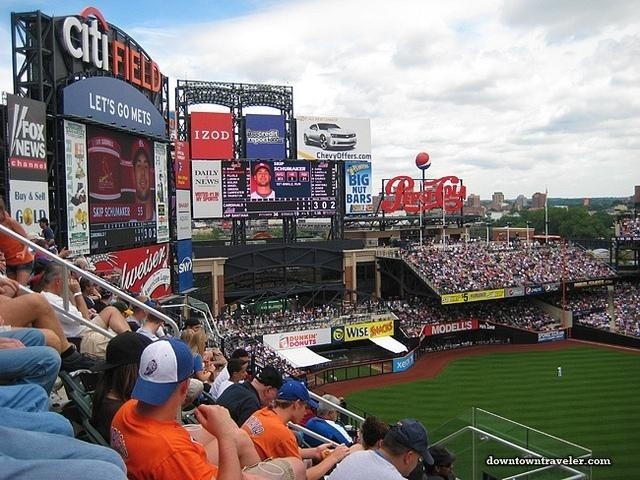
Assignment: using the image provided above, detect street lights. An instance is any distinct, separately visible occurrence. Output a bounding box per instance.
[486,222,493,247]
[526,221,532,248]
[465,222,471,246]
[419,224,426,248]
[506,222,513,248]
[544,219,552,244]
[442,225,448,251]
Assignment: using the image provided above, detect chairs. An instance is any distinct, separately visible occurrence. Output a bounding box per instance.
[53,364,201,451]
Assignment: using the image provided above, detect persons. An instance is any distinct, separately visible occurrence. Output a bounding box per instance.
[0,196,640,480]
[131,138,156,220]
[252,163,276,201]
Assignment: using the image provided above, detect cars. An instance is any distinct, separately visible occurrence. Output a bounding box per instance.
[303,122,357,151]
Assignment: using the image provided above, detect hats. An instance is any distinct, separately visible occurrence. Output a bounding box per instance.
[255,366,319,409]
[390,416,434,466]
[132,297,156,311]
[253,163,271,174]
[427,445,456,464]
[38,217,48,223]
[88,330,154,370]
[131,337,204,407]
[132,138,152,166]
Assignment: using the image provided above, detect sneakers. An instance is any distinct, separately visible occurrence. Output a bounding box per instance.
[63,352,108,372]
[49,376,73,411]
